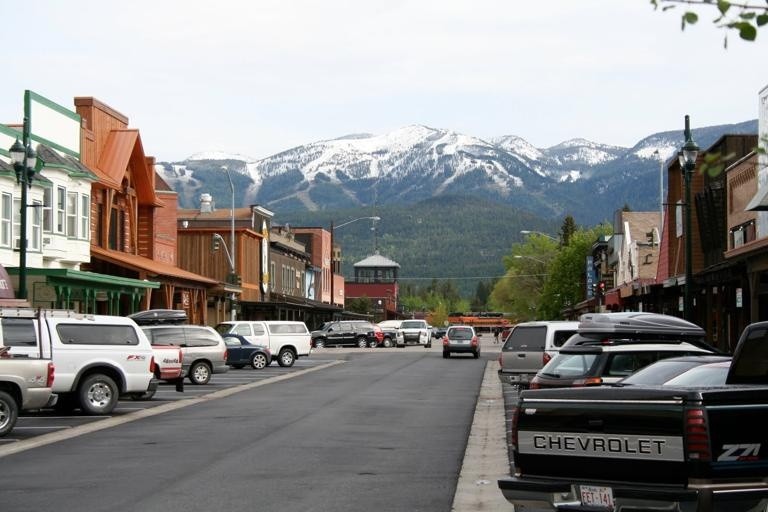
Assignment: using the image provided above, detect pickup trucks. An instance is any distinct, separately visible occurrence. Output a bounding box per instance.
[131,344,186,403]
[497,318,767,512]
[0,357,58,438]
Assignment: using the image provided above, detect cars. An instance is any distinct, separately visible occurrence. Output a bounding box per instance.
[441,325,484,359]
[556,334,632,379]
[661,361,730,389]
[584,356,732,390]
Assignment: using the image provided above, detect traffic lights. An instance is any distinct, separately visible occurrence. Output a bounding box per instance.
[592,283,598,296]
[599,281,607,297]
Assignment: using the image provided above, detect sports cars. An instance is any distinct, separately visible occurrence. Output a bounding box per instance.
[220,333,271,370]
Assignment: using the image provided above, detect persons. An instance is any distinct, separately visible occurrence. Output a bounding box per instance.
[492,328,500,344]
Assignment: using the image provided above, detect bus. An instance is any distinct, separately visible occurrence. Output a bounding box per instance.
[446,312,519,333]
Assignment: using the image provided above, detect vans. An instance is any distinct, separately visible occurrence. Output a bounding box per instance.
[497,320,580,387]
[501,325,513,342]
[126,309,232,386]
[213,321,312,368]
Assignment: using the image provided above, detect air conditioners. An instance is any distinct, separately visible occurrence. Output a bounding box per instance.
[12,237,29,251]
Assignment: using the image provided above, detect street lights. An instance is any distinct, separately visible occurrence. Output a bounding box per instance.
[511,251,546,267]
[518,228,562,247]
[329,215,382,306]
[8,116,47,298]
[211,164,240,321]
[673,111,699,315]
[652,147,666,243]
[373,299,381,322]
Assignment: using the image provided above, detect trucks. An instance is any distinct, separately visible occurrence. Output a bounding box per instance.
[0,297,158,416]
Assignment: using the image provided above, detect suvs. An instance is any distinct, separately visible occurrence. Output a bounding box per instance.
[310,318,447,351]
[524,311,724,393]
